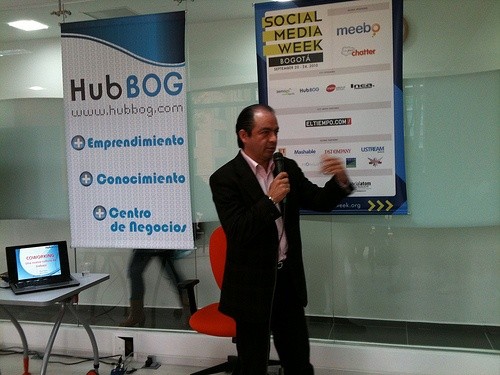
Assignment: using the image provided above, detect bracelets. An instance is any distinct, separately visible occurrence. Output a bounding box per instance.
[269,196,276,206]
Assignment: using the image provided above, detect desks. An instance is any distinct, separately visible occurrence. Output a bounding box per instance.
[0,273,110,375]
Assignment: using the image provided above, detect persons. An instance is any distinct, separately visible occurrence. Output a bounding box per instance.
[118,249,200,327]
[208,102,356,375]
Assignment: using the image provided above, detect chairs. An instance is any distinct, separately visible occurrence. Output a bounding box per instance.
[176,226,284,375]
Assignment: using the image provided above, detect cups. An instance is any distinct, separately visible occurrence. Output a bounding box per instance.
[79,263,90,277]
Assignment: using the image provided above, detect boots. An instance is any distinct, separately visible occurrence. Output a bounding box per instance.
[120,300,146,326]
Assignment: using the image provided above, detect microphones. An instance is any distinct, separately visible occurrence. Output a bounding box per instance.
[273,152,286,203]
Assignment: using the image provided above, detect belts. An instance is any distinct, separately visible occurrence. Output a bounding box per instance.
[278,259,288,269]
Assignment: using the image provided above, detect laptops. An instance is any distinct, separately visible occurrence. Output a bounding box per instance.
[6,241,80,295]
